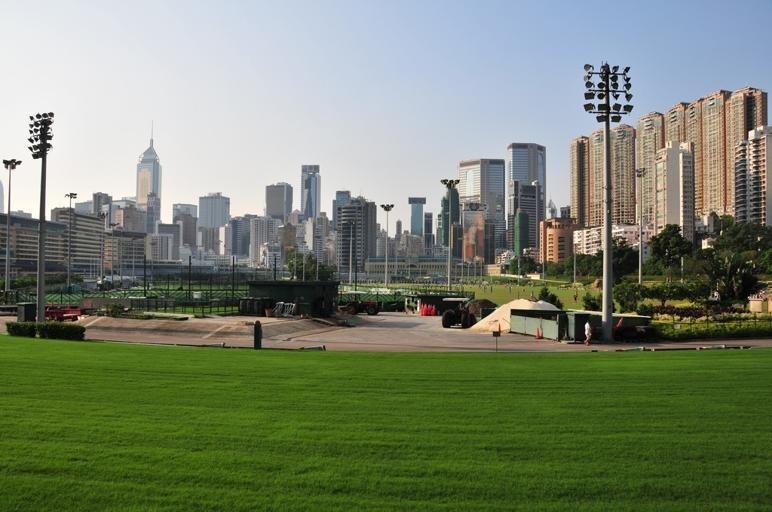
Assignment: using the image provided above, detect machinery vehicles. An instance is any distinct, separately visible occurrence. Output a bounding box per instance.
[336,293,383,315]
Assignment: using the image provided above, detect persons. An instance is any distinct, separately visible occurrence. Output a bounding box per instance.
[584,320,593,346]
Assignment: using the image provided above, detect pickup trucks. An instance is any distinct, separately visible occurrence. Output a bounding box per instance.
[45,304,80,321]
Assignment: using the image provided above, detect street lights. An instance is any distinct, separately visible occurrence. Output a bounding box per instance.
[346,218,356,283]
[440,178,460,290]
[583,61,633,342]
[379,203,394,289]
[64,193,76,286]
[97,211,109,280]
[3,158,22,293]
[27,112,54,322]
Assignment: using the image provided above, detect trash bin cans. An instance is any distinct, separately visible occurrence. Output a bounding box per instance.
[17,301,37,322]
